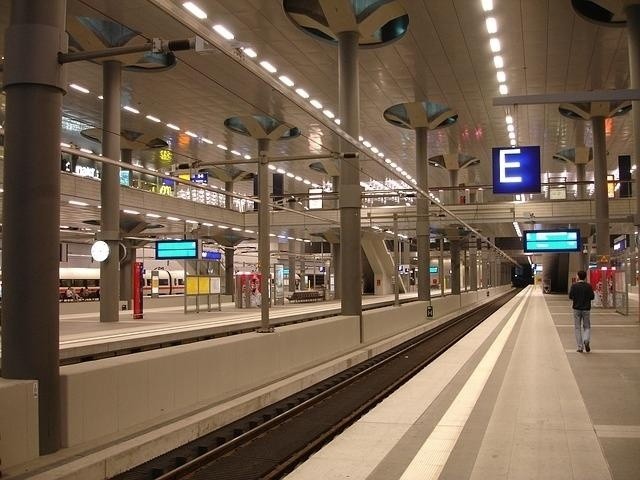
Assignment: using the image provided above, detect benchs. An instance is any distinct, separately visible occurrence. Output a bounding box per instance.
[286,292,318,303]
[61,291,100,303]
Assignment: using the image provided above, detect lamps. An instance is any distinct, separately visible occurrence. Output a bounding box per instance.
[120,243,128,263]
[90,241,111,262]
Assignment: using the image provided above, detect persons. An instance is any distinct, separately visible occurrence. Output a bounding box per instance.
[66,286,100,302]
[68,142,80,172]
[569,271,594,353]
[61,155,67,172]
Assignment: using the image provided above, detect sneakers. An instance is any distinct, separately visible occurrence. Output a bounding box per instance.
[585,340,590,352]
[577,350,583,352]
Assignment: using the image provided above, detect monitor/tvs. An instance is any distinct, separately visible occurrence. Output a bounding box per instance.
[155,239,198,260]
[429,267,438,273]
[120,170,129,186]
[523,229,580,255]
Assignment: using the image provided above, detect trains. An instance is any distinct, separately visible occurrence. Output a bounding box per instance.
[59,267,184,297]
